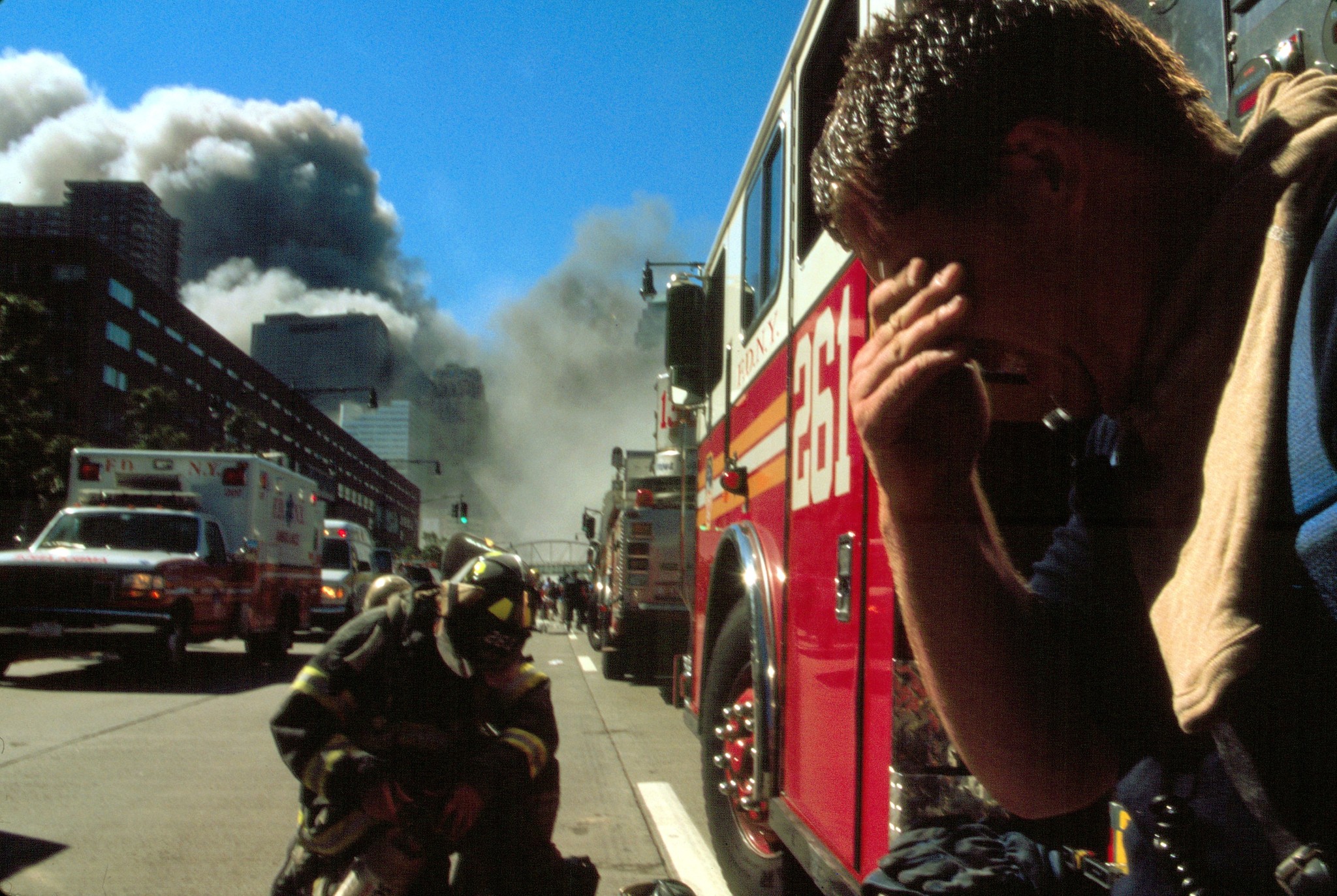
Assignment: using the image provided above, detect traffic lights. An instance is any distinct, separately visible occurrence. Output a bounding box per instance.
[452,505,459,518]
[460,502,468,523]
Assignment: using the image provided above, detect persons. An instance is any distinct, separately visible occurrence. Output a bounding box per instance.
[524,569,590,632]
[809,0,1337,896]
[269,550,560,896]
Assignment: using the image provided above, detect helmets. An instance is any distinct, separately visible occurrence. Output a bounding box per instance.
[437,549,540,678]
[363,574,411,609]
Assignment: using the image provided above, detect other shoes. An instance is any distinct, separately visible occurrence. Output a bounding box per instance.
[455,856,599,895]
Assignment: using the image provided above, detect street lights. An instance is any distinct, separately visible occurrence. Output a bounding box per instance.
[288,387,378,473]
[379,457,442,547]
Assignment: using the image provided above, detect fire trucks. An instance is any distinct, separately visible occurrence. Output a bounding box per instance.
[0,446,324,687]
[660,1,1337,896]
[587,446,697,681]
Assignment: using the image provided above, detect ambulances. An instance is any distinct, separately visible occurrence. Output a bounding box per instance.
[311,518,378,629]
